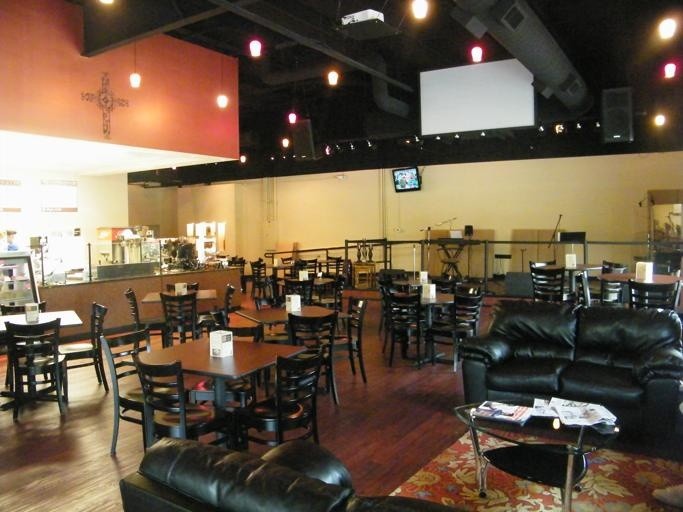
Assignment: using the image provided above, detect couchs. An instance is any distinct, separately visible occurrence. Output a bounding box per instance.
[112,437,468,511]
[456,296,683,457]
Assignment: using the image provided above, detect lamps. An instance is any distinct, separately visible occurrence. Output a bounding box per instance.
[129,39,143,90]
[216,4,228,109]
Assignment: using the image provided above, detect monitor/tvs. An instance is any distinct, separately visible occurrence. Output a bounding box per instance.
[392,166,421,192]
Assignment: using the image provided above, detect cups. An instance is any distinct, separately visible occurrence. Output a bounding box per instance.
[273,258,282,267]
[142,225,149,237]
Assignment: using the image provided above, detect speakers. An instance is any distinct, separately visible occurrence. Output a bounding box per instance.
[290,118,328,161]
[602,86,634,144]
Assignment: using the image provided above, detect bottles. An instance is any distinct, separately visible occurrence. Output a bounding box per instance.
[182,260,190,270]
[205,226,211,237]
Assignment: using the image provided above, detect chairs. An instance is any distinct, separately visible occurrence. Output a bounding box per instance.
[96,321,185,457]
[59,301,112,392]
[286,311,342,405]
[124,225,683,371]
[3,301,49,388]
[322,295,370,381]
[129,360,213,455]
[224,344,326,451]
[3,319,69,422]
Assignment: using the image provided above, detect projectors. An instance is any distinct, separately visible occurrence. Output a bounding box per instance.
[341,8,384,25]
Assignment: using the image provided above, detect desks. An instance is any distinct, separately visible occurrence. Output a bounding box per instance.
[3,310,85,410]
[137,338,307,416]
[451,396,619,511]
